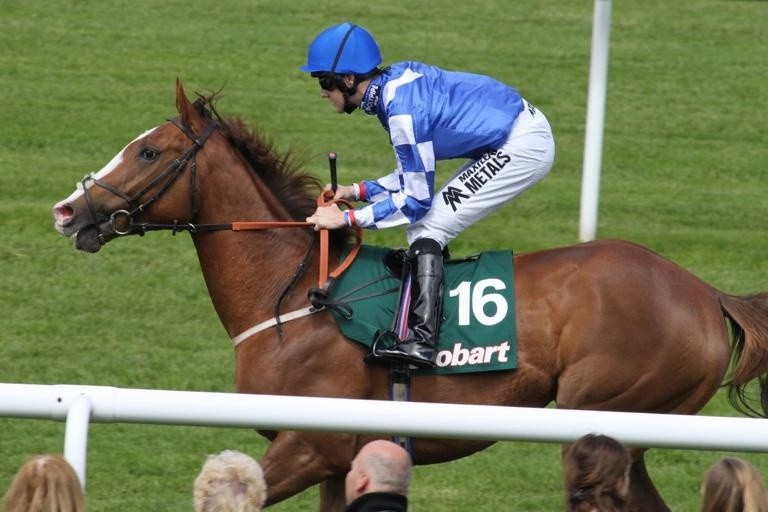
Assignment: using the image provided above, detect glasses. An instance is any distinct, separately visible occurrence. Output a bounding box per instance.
[320,25,352,90]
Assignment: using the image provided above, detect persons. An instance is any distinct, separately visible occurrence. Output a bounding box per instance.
[341,438,412,512]
[2,457,86,512]
[563,433,634,512]
[192,449,268,512]
[298,22,555,371]
[700,457,768,512]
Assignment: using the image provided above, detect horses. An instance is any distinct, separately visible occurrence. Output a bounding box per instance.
[49,74,768,512]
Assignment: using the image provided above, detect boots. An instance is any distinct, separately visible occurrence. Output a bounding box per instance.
[364,238,443,365]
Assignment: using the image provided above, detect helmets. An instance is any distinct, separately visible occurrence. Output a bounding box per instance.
[300,25,381,73]
